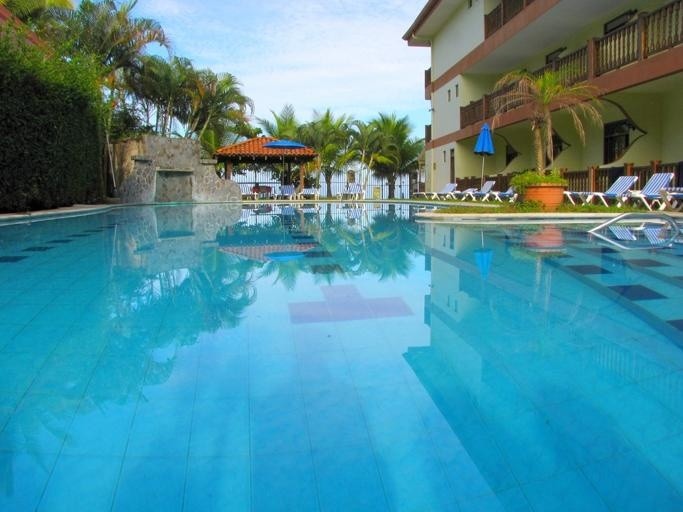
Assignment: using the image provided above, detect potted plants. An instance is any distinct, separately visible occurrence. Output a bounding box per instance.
[509,168,569,212]
[506,223,566,259]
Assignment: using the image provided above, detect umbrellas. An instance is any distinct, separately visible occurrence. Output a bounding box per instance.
[262,205,308,265]
[262,139,304,201]
[472,223,494,286]
[472,123,496,188]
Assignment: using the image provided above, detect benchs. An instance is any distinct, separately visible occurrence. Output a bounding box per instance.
[242,193,258,200]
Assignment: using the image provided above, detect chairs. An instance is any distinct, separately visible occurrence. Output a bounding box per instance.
[607,224,683,247]
[412,180,518,203]
[279,182,363,200]
[279,204,364,219]
[563,172,683,212]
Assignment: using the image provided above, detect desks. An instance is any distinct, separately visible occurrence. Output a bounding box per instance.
[253,204,273,214]
[251,186,271,199]
